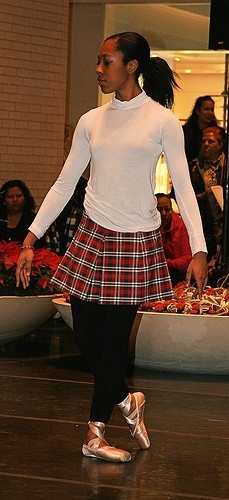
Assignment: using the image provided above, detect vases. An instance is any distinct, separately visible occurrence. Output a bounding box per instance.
[0,293,61,345]
[52,297,229,376]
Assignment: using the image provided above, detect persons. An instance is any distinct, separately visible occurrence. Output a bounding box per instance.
[14,31,207,463]
[0,179,47,287]
[179,94,225,173]
[152,192,193,288]
[185,122,229,276]
[43,176,86,259]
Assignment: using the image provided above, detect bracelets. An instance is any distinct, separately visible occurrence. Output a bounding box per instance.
[20,246,34,250]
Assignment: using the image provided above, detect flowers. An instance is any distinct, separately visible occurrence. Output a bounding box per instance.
[63,282,229,316]
[0,239,63,296]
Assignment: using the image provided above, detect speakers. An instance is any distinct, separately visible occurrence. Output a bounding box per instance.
[208,0,229,51]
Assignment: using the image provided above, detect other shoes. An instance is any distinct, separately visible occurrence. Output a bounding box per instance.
[82,421,132,461]
[118,391,150,449]
